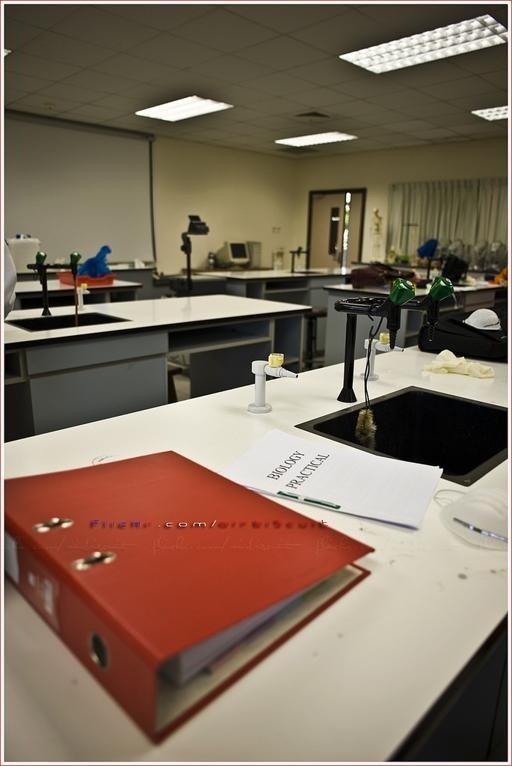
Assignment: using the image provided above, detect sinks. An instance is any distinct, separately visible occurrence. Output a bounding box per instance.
[3,310,135,332]
[294,384,508,488]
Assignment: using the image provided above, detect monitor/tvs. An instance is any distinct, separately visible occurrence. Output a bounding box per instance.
[215,241,250,270]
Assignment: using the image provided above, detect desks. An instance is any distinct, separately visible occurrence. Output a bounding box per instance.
[321,280,505,369]
[14,278,143,308]
[2,294,312,444]
[4,351,510,766]
[192,266,354,348]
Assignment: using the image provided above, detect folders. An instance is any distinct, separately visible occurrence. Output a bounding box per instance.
[4,451,375,749]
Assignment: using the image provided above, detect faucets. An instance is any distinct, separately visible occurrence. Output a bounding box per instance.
[330,273,457,403]
[24,249,87,316]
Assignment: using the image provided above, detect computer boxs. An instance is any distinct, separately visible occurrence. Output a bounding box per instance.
[247,240,262,269]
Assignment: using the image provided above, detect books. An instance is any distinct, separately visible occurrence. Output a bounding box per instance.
[229,427,444,529]
